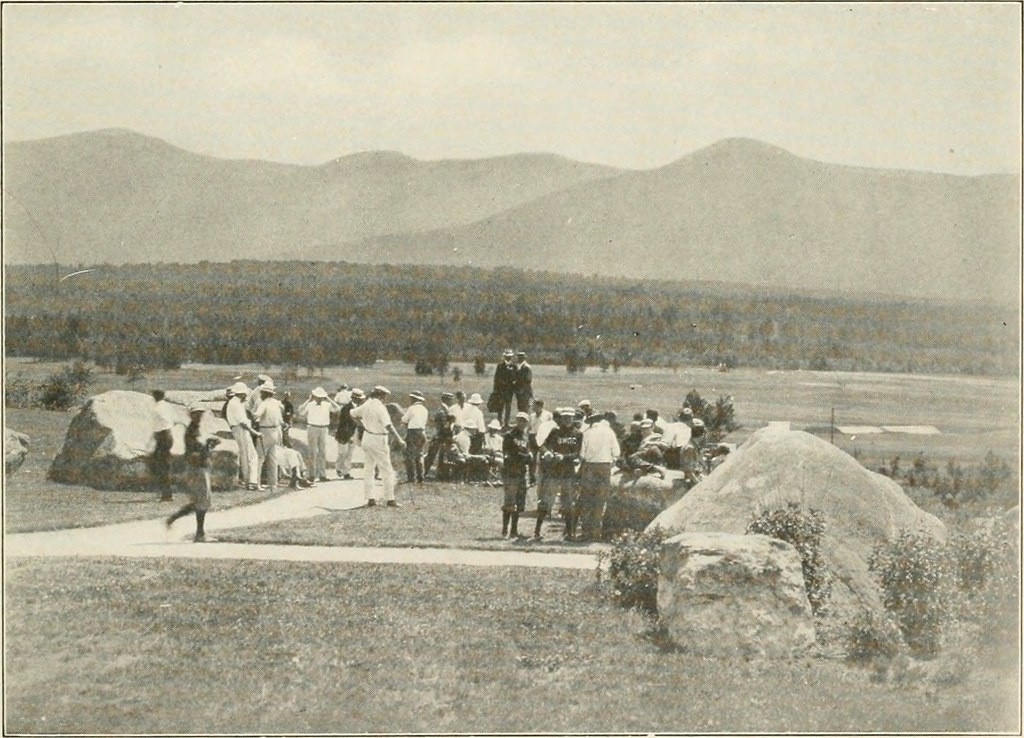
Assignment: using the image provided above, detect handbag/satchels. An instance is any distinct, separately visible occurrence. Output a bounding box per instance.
[540,455,562,478]
[487,391,504,412]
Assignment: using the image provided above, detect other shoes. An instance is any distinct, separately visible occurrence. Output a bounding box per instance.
[369,499,376,505]
[388,501,402,507]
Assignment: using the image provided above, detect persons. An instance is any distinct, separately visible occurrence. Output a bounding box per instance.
[221,371,733,541]
[146,388,176,503]
[493,348,520,427]
[166,401,222,544]
[513,351,533,415]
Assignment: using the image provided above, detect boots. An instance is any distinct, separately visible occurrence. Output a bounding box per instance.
[502,510,510,536]
[194,511,217,542]
[510,511,519,536]
[534,511,548,540]
[166,504,194,529]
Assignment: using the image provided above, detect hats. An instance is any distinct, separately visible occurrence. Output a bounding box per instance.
[488,419,502,430]
[578,400,590,406]
[464,418,476,429]
[560,407,575,416]
[516,412,529,421]
[234,383,247,393]
[584,408,604,424]
[312,387,327,397]
[503,351,514,356]
[468,394,483,404]
[350,389,366,398]
[260,385,276,395]
[640,419,653,427]
[409,390,425,400]
[516,352,527,358]
[188,402,205,412]
[373,386,391,394]
[442,393,453,399]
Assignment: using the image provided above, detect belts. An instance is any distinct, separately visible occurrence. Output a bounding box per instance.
[259,426,276,428]
[311,424,328,428]
[365,429,388,435]
